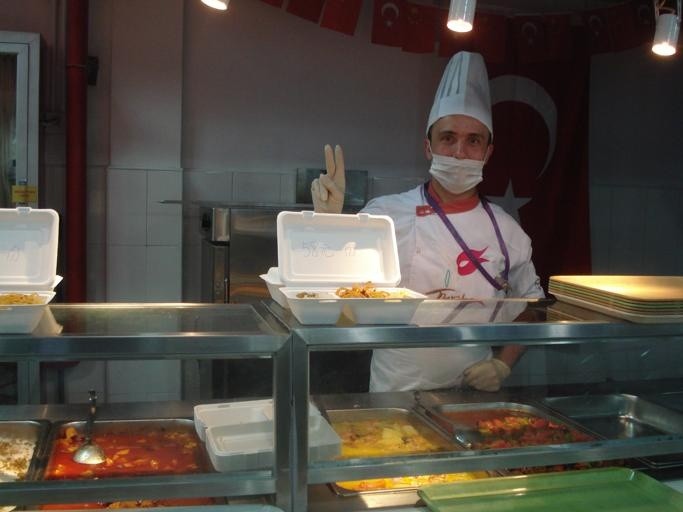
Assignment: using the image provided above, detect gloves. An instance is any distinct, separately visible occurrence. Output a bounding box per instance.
[463,357,512,394]
[311,143,346,214]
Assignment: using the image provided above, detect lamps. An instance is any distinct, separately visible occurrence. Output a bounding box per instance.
[650,0,681,62]
[445,1,476,34]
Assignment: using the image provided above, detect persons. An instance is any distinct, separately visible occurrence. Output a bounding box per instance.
[311,115,546,392]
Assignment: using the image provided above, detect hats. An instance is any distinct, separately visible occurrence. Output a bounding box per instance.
[424,50,494,145]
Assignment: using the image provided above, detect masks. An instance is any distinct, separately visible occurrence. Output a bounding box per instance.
[427,139,490,196]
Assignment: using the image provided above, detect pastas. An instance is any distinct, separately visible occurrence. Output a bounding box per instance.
[0,292,44,304]
[335,284,390,298]
